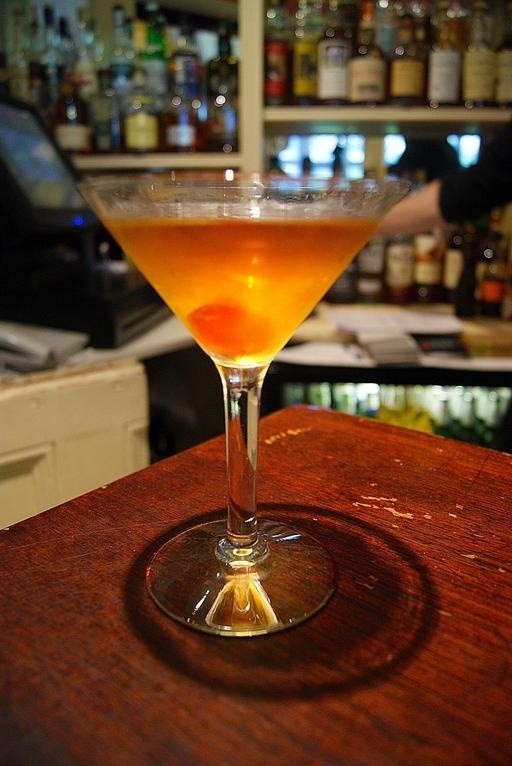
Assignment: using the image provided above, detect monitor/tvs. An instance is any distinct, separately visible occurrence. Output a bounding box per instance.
[0,91,102,242]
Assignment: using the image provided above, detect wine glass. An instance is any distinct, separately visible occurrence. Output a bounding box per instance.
[77,178,412,642]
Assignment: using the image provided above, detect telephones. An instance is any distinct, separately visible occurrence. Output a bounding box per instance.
[1,320,90,375]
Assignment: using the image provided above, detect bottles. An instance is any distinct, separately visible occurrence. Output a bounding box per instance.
[434,388,506,444]
[263,0,512,109]
[325,209,511,322]
[266,146,429,183]
[11,0,240,153]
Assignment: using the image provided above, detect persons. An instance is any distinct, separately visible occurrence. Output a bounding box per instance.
[375,117,509,240]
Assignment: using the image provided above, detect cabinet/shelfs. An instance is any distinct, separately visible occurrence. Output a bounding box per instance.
[1,0,512,392]
[1,345,148,527]
